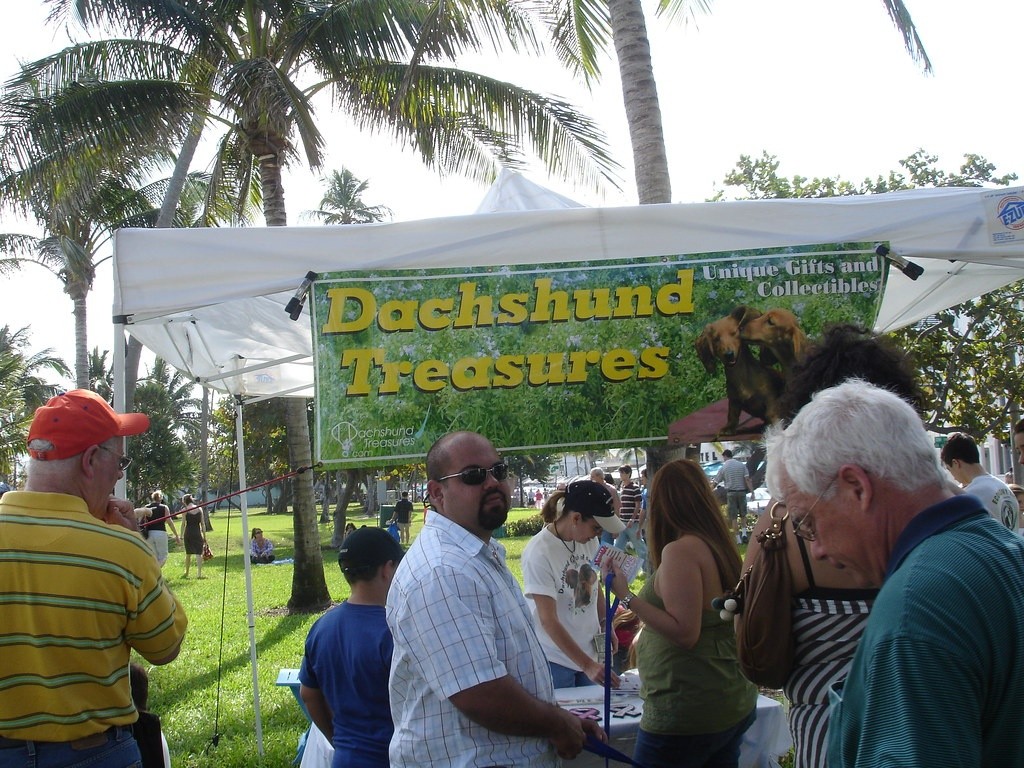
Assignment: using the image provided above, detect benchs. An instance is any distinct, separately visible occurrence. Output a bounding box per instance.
[276,669,313,762]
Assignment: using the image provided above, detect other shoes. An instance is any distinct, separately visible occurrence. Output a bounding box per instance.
[737,540,742,545]
[742,534,748,543]
[401,542,409,549]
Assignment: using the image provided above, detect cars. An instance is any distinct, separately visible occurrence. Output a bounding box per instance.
[512,452,771,518]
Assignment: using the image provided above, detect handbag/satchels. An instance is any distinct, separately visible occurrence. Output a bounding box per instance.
[736,501,793,689]
[139,504,148,539]
[203,542,213,560]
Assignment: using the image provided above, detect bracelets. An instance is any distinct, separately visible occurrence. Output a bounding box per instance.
[621,593,634,610]
[599,617,605,624]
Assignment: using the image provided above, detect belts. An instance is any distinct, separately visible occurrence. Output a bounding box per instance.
[728,490,746,492]
[0,725,128,749]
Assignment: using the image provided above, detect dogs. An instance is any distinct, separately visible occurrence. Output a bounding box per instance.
[693,304,818,436]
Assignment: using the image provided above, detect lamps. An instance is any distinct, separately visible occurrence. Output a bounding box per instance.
[875,243,924,281]
[285,270,317,321]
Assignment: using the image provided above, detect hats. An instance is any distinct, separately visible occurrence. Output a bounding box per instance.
[564,481,627,533]
[26,389,149,461]
[338,525,406,573]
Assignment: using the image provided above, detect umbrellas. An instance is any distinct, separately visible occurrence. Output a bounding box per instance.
[668,398,767,445]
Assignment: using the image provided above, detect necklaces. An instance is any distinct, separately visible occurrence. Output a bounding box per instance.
[555,523,575,558]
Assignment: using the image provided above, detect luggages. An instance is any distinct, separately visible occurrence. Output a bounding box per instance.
[386,519,400,544]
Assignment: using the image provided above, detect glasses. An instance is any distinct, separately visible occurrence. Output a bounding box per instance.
[423,464,509,502]
[81,444,133,470]
[255,531,263,534]
[793,468,869,542]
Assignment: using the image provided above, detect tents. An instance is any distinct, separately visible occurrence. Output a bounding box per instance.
[111,172,1024,750]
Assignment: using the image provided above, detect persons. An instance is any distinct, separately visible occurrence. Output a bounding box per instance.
[711,450,755,545]
[590,464,648,573]
[602,460,758,768]
[180,494,207,580]
[386,430,608,767]
[346,523,356,534]
[519,480,621,688]
[733,322,1024,768]
[130,664,172,768]
[528,488,549,509]
[134,491,180,568]
[298,525,406,768]
[686,448,699,461]
[0,388,189,768]
[391,492,413,544]
[251,528,275,563]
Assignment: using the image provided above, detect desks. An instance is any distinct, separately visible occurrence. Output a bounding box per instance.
[300,679,793,768]
